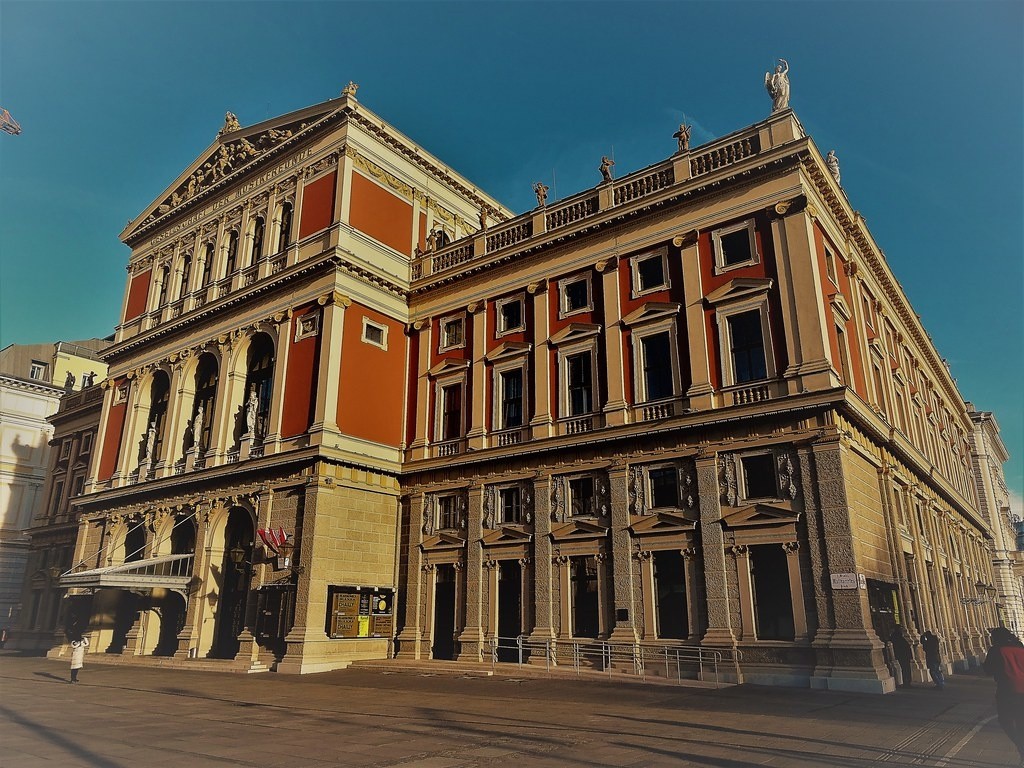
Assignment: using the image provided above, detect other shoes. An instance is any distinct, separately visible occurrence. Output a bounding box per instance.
[903,685,915,689]
[72,680,79,684]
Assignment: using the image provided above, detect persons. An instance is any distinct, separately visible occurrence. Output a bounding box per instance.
[890,624,912,688]
[828,149,840,187]
[477,207,490,229]
[0,629,8,649]
[673,125,690,150]
[246,392,259,433]
[772,58,790,108]
[920,631,944,688]
[87,371,96,385]
[983,628,1024,752]
[70,635,89,684]
[342,80,359,94]
[192,406,206,446]
[146,422,159,460]
[427,229,440,251]
[535,183,549,205]
[599,156,614,181]
